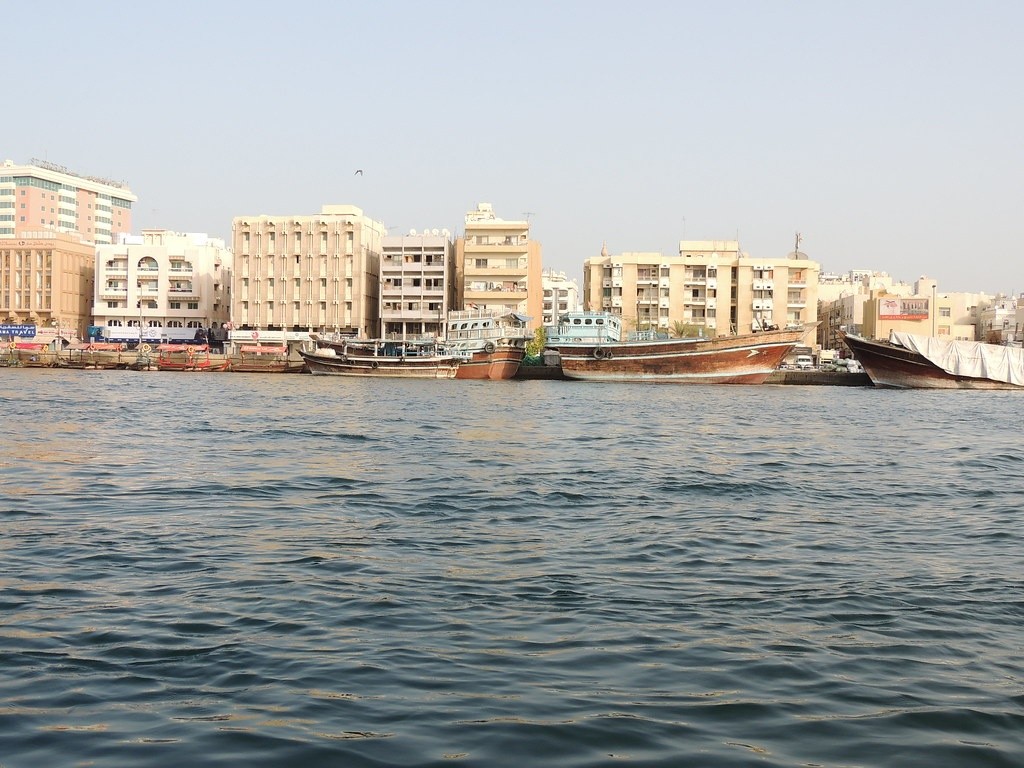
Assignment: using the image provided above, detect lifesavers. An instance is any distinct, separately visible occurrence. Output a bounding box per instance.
[341,354,348,362]
[606,352,613,358]
[350,360,356,365]
[592,347,606,360]
[9,342,16,349]
[90,343,95,349]
[188,346,194,353]
[400,357,405,363]
[43,344,49,351]
[143,344,152,352]
[484,341,496,354]
[371,361,379,369]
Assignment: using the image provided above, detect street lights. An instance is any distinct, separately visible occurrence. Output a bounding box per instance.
[932,283,937,336]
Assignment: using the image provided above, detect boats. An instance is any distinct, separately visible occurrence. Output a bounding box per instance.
[63,337,128,368]
[299,310,533,381]
[129,348,160,372]
[1,337,56,369]
[229,343,305,375]
[155,343,233,372]
[832,329,1024,391]
[540,311,824,386]
